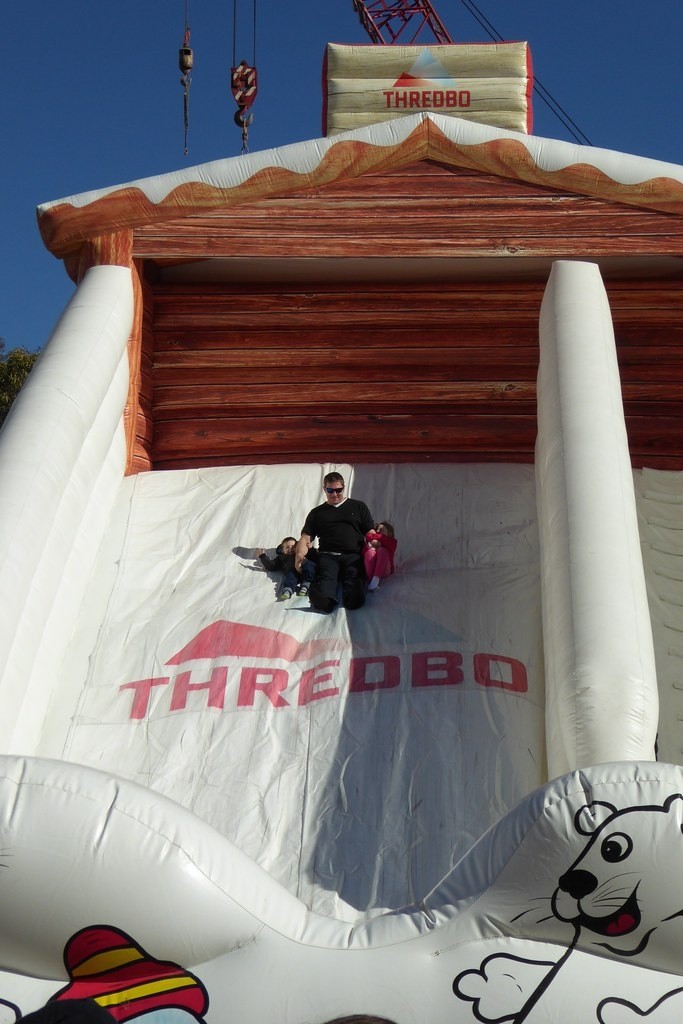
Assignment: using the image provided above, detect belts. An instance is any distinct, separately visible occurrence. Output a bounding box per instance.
[318,551,342,556]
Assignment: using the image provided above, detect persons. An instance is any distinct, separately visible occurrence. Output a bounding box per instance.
[362,521,397,593]
[294,472,379,613]
[255,537,319,600]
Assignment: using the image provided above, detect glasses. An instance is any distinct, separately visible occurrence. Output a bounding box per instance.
[326,487,343,493]
